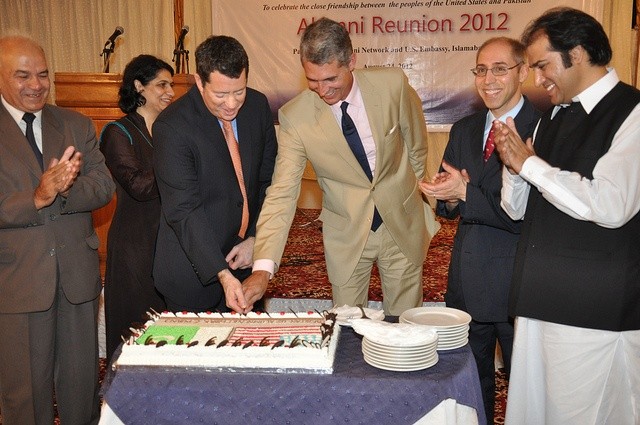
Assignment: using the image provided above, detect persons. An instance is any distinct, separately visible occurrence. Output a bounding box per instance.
[490,5,640,425]
[235,18,442,318]
[418,36,544,425]
[99,54,175,371]
[0,35,117,425]
[151,36,279,315]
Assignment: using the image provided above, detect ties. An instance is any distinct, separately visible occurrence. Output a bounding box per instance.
[483,121,496,162]
[340,102,383,232]
[221,121,249,238]
[23,113,43,171]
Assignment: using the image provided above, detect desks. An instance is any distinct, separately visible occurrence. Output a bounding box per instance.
[97,314,486,425]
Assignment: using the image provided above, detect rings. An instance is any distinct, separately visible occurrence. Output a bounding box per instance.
[434,194,435,198]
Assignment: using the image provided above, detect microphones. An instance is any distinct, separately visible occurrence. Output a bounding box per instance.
[105,25,124,46]
[176,25,190,49]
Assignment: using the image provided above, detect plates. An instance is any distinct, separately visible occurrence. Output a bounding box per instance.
[361,324,438,372]
[399,305,473,351]
[326,302,385,326]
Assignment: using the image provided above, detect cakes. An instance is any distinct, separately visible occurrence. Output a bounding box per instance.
[116,305,340,367]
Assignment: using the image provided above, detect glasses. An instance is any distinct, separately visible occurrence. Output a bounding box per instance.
[470,62,522,77]
